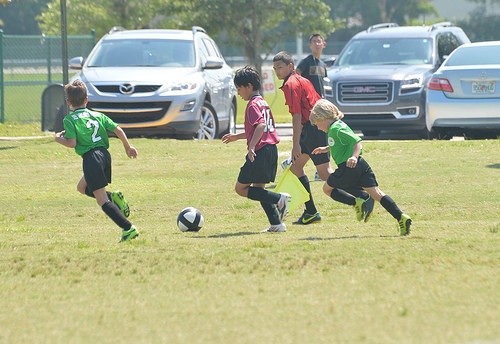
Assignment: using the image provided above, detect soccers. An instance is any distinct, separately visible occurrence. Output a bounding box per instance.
[177,207,204,232]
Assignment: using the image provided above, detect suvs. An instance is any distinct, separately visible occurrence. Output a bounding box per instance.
[325,20,472,144]
[67,25,240,141]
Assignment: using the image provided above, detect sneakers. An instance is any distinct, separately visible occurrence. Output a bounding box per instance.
[263,222,286,232]
[276,193,292,221]
[314,172,322,181]
[397,214,412,236]
[292,209,322,226]
[363,194,375,222]
[110,191,130,218]
[281,160,290,169]
[353,198,367,221]
[117,225,139,243]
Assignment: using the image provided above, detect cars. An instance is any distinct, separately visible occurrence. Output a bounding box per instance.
[424,39,500,139]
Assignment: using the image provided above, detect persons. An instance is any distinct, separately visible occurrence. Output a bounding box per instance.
[55,80,139,242]
[280,34,327,180]
[310,98,412,236]
[273,51,364,225]
[222,67,291,233]
